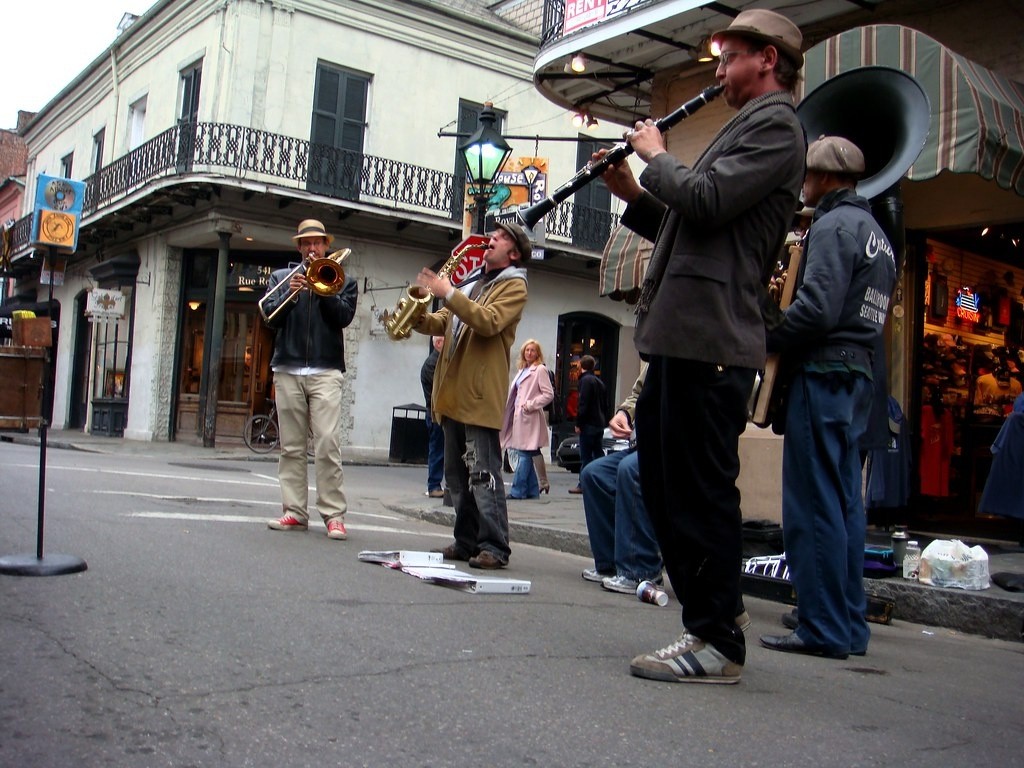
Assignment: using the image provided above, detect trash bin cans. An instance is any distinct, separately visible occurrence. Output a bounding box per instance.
[387,404,431,462]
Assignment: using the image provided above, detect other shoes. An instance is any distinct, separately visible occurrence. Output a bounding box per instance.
[630,610,751,684]
[506,494,539,499]
[782,608,800,629]
[429,490,444,497]
[568,488,582,493]
[469,552,506,569]
[430,543,480,561]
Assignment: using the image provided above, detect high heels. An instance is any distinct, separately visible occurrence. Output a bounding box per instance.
[539,478,549,494]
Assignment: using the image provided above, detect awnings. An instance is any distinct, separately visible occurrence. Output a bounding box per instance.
[790,21,1024,200]
[598,224,655,305]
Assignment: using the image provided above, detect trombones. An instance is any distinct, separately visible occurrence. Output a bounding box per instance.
[258,247,353,325]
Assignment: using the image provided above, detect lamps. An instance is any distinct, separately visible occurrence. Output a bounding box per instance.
[688,29,721,62]
[565,51,591,75]
[571,102,599,131]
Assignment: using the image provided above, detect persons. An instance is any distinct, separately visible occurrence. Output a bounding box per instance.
[579,364,665,596]
[400,222,530,570]
[507,339,556,500]
[568,355,609,494]
[421,336,445,498]
[757,134,896,659]
[263,220,358,542]
[589,9,809,686]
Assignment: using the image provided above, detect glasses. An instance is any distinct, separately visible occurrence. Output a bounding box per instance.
[721,51,742,66]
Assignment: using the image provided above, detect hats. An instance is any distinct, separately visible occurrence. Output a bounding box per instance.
[806,134,865,172]
[292,220,334,251]
[711,8,804,70]
[494,221,530,262]
[923,333,1024,403]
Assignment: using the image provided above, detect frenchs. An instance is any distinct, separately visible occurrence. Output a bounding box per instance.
[752,66,935,428]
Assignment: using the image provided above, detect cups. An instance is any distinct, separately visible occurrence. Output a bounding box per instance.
[635,578,668,607]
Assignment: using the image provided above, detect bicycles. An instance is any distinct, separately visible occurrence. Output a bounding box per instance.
[243,398,316,458]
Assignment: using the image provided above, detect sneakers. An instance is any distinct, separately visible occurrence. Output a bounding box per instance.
[328,521,347,539]
[268,514,308,531]
[582,569,664,594]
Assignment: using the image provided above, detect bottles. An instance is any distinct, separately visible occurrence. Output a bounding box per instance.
[903,540,921,580]
[891,526,909,564]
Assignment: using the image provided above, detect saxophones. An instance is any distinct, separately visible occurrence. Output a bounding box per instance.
[513,81,726,232]
[376,240,495,343]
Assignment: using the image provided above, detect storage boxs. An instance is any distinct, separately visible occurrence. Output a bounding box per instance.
[13,317,52,347]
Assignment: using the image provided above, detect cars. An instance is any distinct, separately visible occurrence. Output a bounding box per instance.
[556,427,629,474]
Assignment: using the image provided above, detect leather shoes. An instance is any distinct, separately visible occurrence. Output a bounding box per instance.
[759,631,866,659]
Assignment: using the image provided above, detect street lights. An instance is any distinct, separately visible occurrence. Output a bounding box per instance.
[460,105,513,236]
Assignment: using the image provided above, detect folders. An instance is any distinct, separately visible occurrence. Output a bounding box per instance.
[432,577,530,593]
[358,550,445,567]
